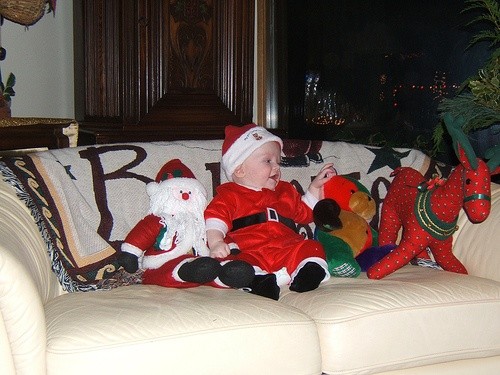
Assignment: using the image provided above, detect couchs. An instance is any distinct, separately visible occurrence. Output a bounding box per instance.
[0,139,500,375]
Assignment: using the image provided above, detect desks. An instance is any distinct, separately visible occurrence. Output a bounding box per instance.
[0,118,77,150]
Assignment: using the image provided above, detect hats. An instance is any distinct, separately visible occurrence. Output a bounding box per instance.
[222,124,284,182]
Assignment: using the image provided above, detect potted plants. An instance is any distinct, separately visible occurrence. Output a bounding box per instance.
[0,70,16,117]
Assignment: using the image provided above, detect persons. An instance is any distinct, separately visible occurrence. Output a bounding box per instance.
[204,125,336,300]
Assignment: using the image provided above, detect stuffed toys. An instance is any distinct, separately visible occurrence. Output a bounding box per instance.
[313,112,500,279]
[120,159,255,290]
